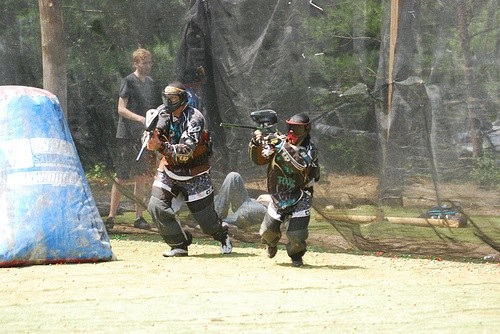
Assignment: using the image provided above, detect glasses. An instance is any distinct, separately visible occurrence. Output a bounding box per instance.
[285,120,308,137]
[161,91,182,105]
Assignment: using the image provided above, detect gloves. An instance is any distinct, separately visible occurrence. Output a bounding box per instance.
[252,129,263,146]
[146,131,160,150]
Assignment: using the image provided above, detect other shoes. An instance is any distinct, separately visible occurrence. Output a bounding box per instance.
[291,253,302,267]
[268,234,280,257]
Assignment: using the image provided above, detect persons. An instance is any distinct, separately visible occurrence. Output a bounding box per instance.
[103,49,156,230]
[146,82,232,257]
[247,113,319,266]
[172,65,207,213]
[215,172,271,228]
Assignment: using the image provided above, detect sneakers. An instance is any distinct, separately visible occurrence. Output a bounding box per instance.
[105,216,114,229]
[134,217,150,229]
[220,234,232,255]
[163,247,188,257]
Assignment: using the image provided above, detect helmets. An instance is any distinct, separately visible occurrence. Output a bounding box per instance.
[289,113,312,139]
[162,81,189,113]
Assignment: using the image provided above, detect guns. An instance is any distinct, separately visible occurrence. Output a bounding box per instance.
[136,108,159,162]
[220,109,278,134]
